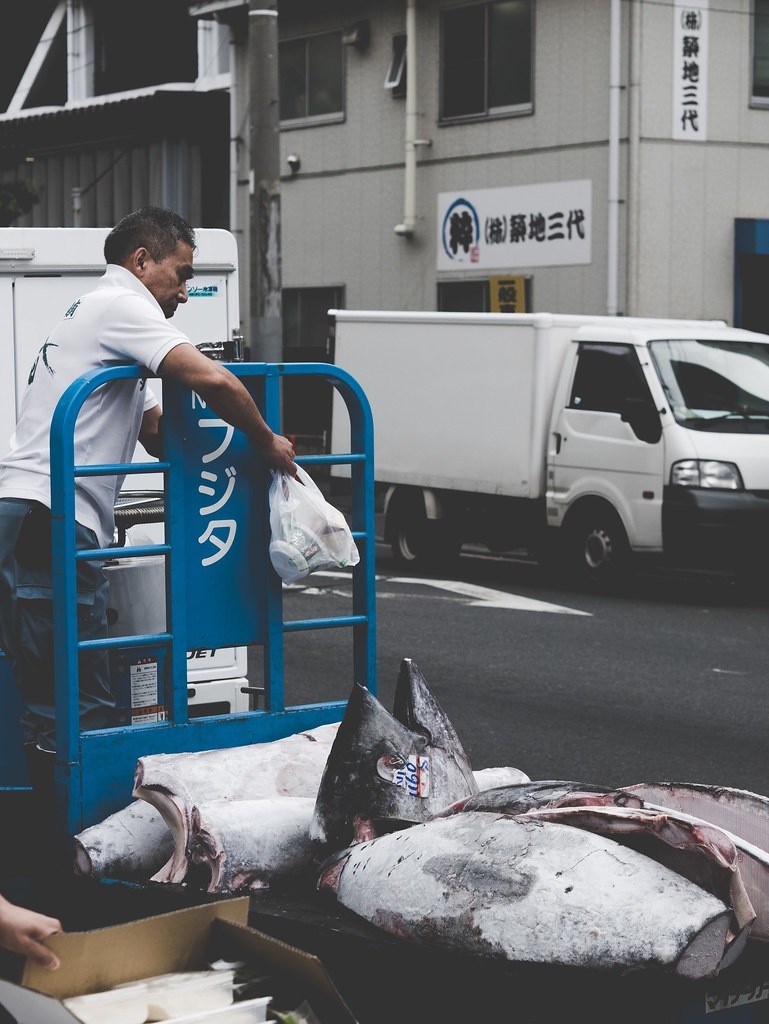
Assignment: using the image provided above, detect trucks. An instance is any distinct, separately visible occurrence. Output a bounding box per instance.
[0,227,259,728]
[325,309,769,595]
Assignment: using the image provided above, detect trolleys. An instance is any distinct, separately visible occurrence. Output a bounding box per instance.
[50,347,769,1024]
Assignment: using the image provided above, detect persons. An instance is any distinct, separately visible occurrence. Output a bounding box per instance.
[0,894,63,971]
[2,206,297,804]
